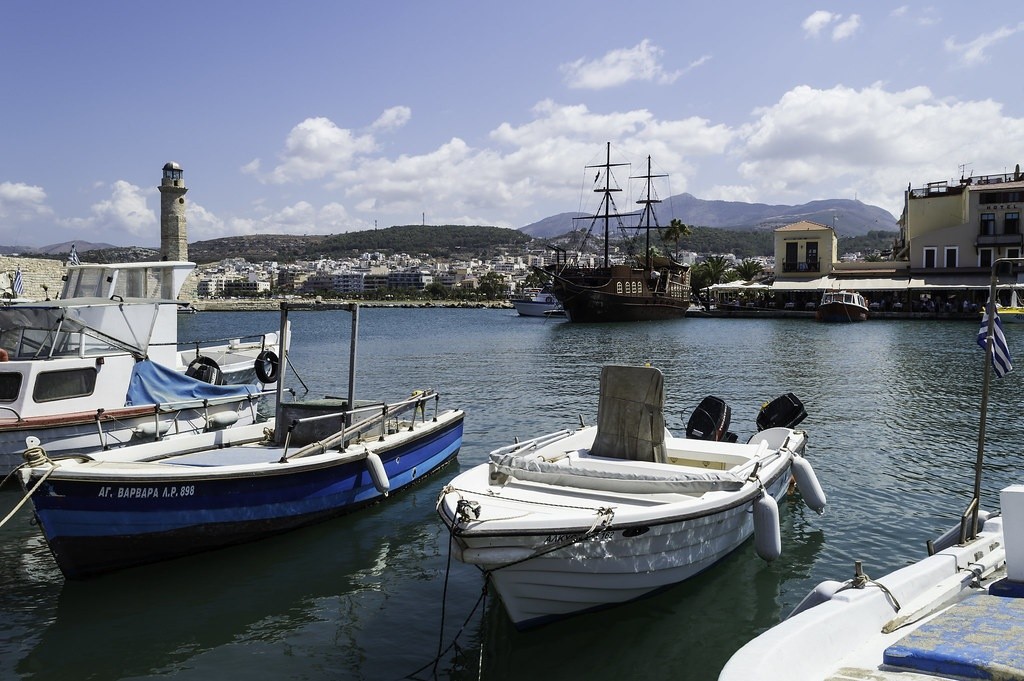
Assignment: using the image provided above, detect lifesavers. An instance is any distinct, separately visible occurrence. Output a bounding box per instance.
[254,350,279,384]
[184,356,224,386]
[546,297,552,303]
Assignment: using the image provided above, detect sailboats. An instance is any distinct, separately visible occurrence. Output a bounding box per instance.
[529,141,692,324]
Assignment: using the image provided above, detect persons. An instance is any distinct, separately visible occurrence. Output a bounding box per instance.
[921,294,951,311]
[964,299,969,312]
[880,299,886,310]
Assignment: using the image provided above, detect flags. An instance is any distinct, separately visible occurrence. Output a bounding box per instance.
[14,271,24,295]
[68,244,82,266]
[975,297,1013,377]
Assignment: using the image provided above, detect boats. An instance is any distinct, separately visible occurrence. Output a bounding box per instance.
[507,284,566,320]
[435,360,811,632]
[12,297,466,580]
[0,258,296,480]
[718,263,1024,681]
[815,285,870,323]
[978,282,1024,326]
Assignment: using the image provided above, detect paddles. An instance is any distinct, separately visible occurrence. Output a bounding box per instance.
[287,388,435,461]
